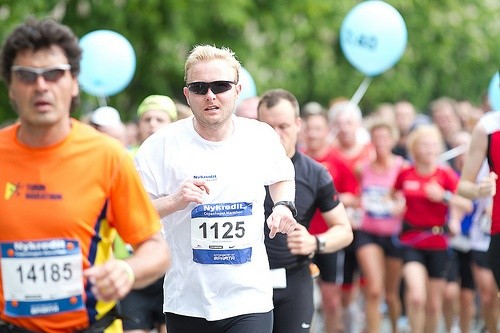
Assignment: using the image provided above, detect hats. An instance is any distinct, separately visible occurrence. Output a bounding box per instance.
[138,95,177,122]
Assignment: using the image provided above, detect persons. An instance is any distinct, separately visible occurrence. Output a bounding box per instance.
[90,95,193,333]
[136,45,297,333]
[258,89,353,333]
[299,96,485,333]
[457,110,500,333]
[235,97,261,120]
[0,18,172,333]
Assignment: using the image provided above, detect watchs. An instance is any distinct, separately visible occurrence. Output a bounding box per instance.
[274,200,297,217]
[315,234,325,252]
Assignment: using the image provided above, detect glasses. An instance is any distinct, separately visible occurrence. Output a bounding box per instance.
[10,64,71,83]
[186,81,237,95]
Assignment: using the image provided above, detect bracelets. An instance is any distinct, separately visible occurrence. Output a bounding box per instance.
[121,260,135,286]
[444,191,452,202]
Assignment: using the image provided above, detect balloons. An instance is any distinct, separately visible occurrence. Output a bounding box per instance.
[340,0,408,78]
[77,29,136,98]
[234,64,256,98]
